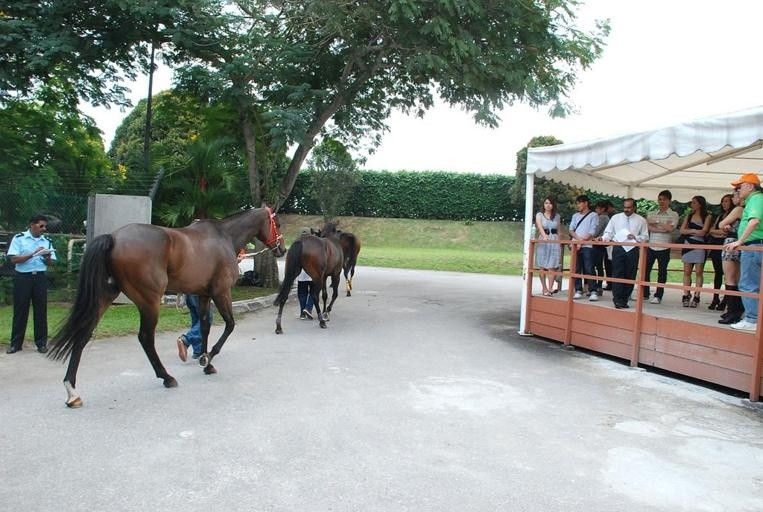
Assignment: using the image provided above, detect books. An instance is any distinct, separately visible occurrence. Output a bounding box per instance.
[32,249,55,257]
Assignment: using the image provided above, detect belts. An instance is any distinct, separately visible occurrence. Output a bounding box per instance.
[740,240,763,247]
[32,271,37,275]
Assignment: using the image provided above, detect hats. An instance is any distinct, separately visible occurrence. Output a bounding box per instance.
[731,174,760,185]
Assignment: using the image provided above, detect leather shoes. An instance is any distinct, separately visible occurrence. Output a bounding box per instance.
[617,305,629,309]
[543,289,558,296]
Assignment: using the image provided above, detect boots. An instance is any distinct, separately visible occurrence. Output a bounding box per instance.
[708,285,744,324]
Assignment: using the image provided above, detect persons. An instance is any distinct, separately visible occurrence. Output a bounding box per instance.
[534,195,562,298]
[175,254,244,363]
[568,172,762,332]
[293,227,315,320]
[5,214,60,354]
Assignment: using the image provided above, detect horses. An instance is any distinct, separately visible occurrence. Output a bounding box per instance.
[44,199,287,408]
[273,215,345,334]
[310,227,361,296]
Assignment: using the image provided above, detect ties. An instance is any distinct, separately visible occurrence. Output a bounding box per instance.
[625,217,629,229]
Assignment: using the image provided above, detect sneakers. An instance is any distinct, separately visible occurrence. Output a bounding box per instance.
[38,345,48,353]
[730,320,757,331]
[573,284,611,300]
[178,337,187,362]
[301,308,314,320]
[7,346,22,354]
[683,295,700,308]
[650,297,660,304]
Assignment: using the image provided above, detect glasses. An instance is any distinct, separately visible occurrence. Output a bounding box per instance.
[35,223,48,228]
